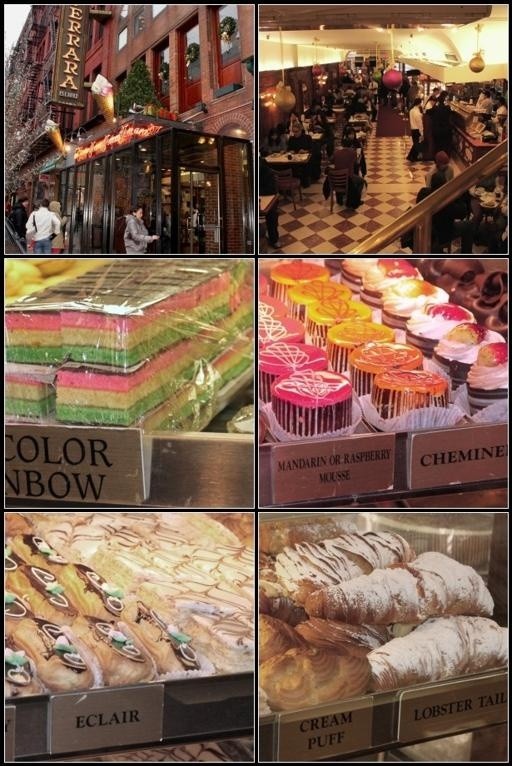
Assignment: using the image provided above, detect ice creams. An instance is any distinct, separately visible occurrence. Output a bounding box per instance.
[91,73,115,131]
[44,119,67,160]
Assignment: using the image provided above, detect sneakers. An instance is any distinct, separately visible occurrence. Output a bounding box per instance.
[353,200,364,209]
[273,241,281,248]
[406,155,418,162]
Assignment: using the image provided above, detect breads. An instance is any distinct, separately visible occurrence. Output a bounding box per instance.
[260,515,508,712]
[4,513,257,696]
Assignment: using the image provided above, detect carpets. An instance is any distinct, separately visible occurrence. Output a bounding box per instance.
[375,97,411,137]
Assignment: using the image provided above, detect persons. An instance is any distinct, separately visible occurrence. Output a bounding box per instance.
[259,156,282,248]
[12,197,30,251]
[381,75,507,254]
[123,205,162,254]
[49,201,68,254]
[259,72,379,208]
[25,198,61,254]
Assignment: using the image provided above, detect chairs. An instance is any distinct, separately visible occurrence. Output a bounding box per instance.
[258,82,368,213]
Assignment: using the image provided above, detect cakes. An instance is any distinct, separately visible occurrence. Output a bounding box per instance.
[259,259,507,437]
[4,257,255,431]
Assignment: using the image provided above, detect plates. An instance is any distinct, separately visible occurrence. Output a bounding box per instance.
[473,187,503,210]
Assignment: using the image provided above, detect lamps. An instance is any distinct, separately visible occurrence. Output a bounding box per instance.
[129,102,144,115]
[64,127,88,145]
